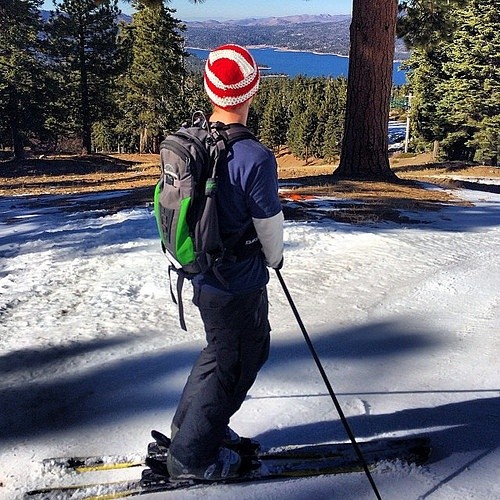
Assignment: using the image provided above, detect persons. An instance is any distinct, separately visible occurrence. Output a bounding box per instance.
[164,42,285,481]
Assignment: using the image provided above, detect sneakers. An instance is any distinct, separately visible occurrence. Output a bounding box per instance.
[171,423,262,458]
[166,443,254,480]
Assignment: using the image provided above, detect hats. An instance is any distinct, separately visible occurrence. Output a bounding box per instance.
[203,43,260,111]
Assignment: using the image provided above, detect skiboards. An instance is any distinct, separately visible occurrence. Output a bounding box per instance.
[24,433,433,500]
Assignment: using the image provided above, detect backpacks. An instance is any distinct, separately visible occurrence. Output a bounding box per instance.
[153,109,261,281]
